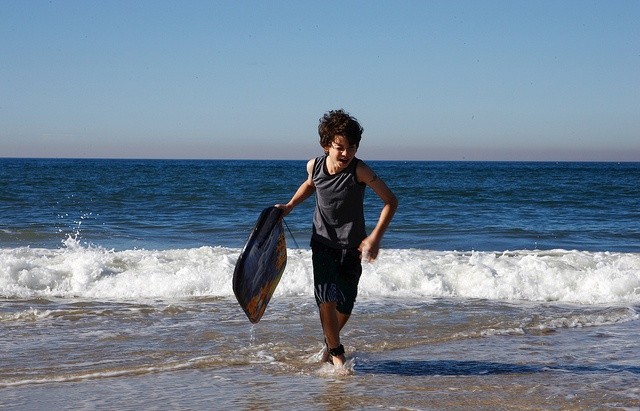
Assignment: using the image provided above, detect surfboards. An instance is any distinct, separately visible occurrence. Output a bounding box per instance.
[232,208,287,325]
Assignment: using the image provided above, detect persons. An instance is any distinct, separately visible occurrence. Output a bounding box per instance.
[273,108,400,370]
